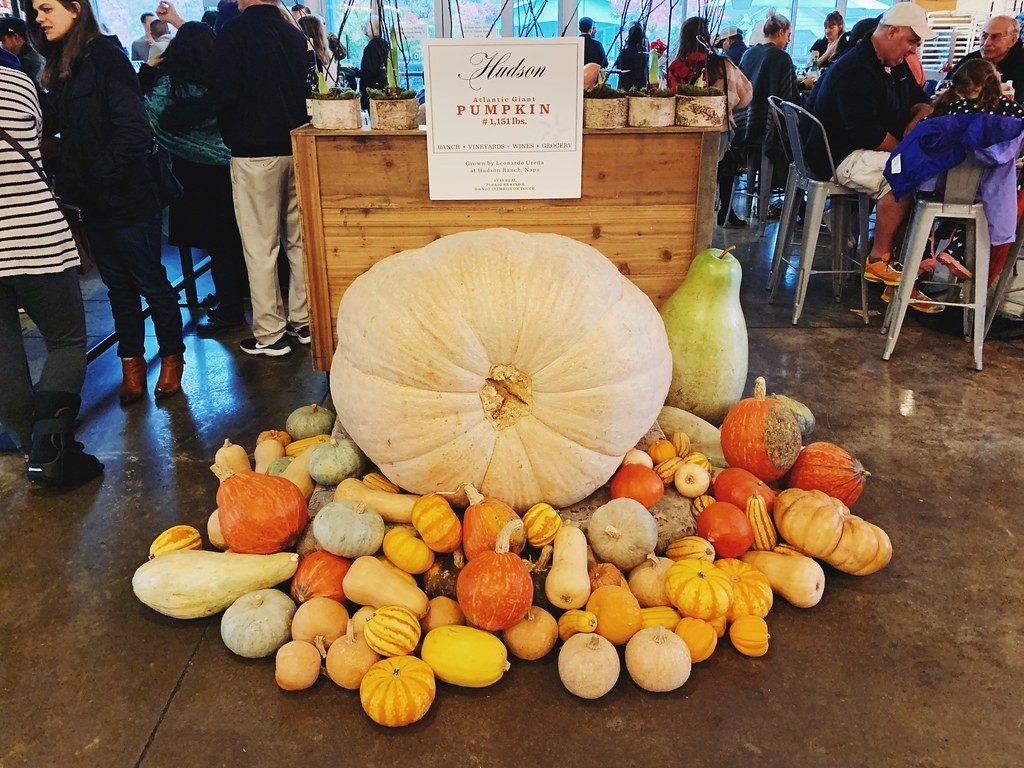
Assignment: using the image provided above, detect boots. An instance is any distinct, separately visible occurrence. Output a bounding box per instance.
[718,175,747,227]
[28,391,104,484]
[936,222,972,281]
[917,220,937,277]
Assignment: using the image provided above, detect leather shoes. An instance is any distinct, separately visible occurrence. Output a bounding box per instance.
[196,316,243,335]
[206,309,221,321]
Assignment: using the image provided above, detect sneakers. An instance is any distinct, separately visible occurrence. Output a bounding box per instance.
[880,284,946,313]
[286,323,311,344]
[239,337,292,357]
[863,255,919,286]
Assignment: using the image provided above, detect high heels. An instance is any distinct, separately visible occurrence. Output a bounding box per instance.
[119,355,148,403]
[155,351,186,399]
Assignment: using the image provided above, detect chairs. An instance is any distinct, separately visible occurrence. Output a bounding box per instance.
[768,99,872,326]
[982,207,1024,344]
[765,95,855,298]
[722,140,790,238]
[880,150,991,371]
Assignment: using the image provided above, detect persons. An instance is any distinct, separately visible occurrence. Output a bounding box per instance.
[1,0,1024,319]
[1,50,106,491]
[18,0,193,408]
[185,0,315,357]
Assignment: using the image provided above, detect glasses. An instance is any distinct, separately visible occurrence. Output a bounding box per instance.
[981,33,1013,41]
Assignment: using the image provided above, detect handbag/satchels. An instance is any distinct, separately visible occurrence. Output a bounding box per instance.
[150,140,186,206]
[57,202,98,275]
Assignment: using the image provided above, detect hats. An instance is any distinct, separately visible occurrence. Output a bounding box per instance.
[714,27,743,48]
[880,1,937,42]
[0,13,26,41]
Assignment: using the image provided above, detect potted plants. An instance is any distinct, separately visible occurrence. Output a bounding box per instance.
[674,83,727,127]
[366,86,420,130]
[626,84,677,127]
[310,87,363,130]
[583,83,629,128]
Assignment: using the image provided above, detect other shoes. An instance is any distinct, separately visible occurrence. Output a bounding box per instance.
[823,209,857,248]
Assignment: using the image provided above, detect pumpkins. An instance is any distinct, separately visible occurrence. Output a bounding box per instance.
[327,227,673,510]
[558,633,620,700]
[359,656,436,728]
[286,404,335,441]
[131,549,300,620]
[545,378,892,663]
[148,525,202,561]
[207,430,563,691]
[624,623,691,693]
